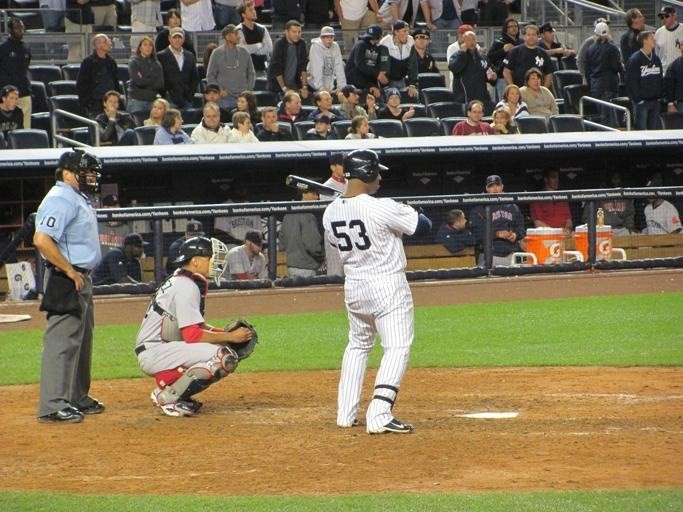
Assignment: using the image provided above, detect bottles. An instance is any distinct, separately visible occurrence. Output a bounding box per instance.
[597,208,603,227]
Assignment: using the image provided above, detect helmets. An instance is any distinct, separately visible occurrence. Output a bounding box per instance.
[58,145,102,194]
[173,236,229,288]
[343,150,389,182]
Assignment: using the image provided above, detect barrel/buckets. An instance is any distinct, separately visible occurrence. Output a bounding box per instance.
[523,226,569,266]
[573,224,614,262]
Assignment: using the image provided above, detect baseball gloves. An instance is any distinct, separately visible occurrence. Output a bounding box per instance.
[224,319,257,360]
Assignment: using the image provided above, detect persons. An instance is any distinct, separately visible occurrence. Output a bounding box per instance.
[134,237,250,416]
[30,153,104,423]
[0,0,683,144]
[93,195,150,281]
[322,149,432,432]
[166,153,349,281]
[438,167,682,267]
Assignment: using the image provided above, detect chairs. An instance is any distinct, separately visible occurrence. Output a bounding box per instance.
[0,0,682,148]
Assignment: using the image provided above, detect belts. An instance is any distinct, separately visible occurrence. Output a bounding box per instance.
[72,265,92,274]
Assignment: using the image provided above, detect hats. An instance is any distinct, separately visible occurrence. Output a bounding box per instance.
[385,87,399,102]
[341,84,362,94]
[363,25,381,38]
[246,230,264,244]
[103,194,121,205]
[321,26,335,36]
[0,85,16,99]
[124,233,150,247]
[486,175,501,187]
[222,24,243,36]
[330,153,343,164]
[413,29,430,39]
[169,26,185,39]
[313,113,329,123]
[539,22,555,33]
[186,221,204,235]
[392,19,408,32]
[658,6,675,15]
[594,18,610,35]
[206,84,219,95]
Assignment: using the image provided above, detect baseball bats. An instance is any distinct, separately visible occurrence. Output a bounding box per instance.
[286,174,424,214]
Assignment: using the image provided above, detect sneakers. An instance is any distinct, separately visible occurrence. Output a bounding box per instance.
[373,418,412,434]
[79,399,104,414]
[150,389,203,417]
[39,406,84,423]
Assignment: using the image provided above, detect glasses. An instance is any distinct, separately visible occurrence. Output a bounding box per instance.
[660,14,670,19]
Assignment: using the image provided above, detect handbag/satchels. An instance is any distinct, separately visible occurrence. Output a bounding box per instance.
[480,54,499,85]
